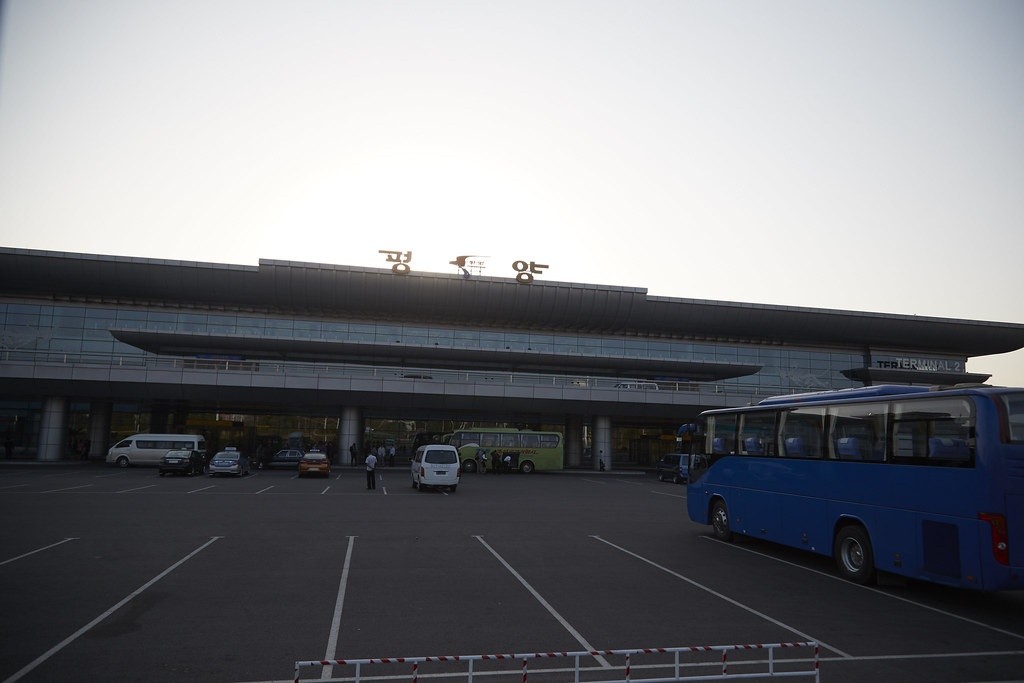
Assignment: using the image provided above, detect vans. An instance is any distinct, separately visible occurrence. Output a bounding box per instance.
[105,433,206,469]
[408,445,461,493]
[655,454,689,484]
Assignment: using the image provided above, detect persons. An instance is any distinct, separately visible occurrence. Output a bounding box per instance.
[475,448,488,473]
[20,432,34,456]
[490,449,511,472]
[68,438,90,456]
[4,437,15,459]
[256,441,396,469]
[365,450,378,489]
[599,450,605,471]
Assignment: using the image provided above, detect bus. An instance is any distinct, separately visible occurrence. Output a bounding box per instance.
[674,384,1024,591]
[441,427,564,474]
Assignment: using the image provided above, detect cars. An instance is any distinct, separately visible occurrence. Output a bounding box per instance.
[299,449,332,479]
[158,448,205,477]
[209,447,250,478]
[271,450,302,462]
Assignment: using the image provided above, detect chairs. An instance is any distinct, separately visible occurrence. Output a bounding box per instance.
[837,437,862,460]
[883,434,913,460]
[785,437,806,457]
[515,440,557,448]
[712,437,729,453]
[745,438,764,455]
[929,438,966,458]
[493,440,500,447]
[482,439,492,446]
[505,440,512,446]
